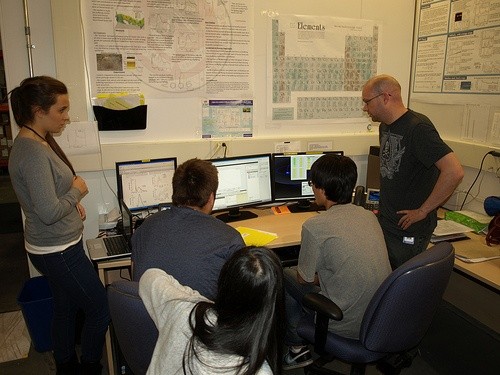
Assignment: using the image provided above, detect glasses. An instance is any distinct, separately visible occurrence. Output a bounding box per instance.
[308,178,315,186]
[363,93,392,105]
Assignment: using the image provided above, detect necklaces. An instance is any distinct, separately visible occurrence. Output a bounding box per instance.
[22,125,48,142]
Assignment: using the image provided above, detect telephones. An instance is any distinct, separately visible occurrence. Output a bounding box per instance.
[352,185,382,215]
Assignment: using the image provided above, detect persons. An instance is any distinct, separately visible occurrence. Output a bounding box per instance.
[6,76,110,374]
[131,159,246,302]
[362,74,465,271]
[138,245,287,375]
[279,153,394,371]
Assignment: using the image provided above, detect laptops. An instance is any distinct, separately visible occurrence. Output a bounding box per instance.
[85,198,136,262]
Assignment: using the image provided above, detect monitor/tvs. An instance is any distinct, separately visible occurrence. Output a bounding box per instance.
[113,156,178,217]
[202,155,273,222]
[271,150,343,214]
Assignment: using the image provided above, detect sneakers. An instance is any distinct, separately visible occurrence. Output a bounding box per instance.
[277,345,314,370]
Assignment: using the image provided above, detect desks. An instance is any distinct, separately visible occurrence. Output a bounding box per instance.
[425,206,500,342]
[95,202,328,375]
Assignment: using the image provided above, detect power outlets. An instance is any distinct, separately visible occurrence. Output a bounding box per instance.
[488,148,500,173]
[210,140,229,160]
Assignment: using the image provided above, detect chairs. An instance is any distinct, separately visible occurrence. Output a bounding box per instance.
[106,280,161,374]
[282,242,455,375]
